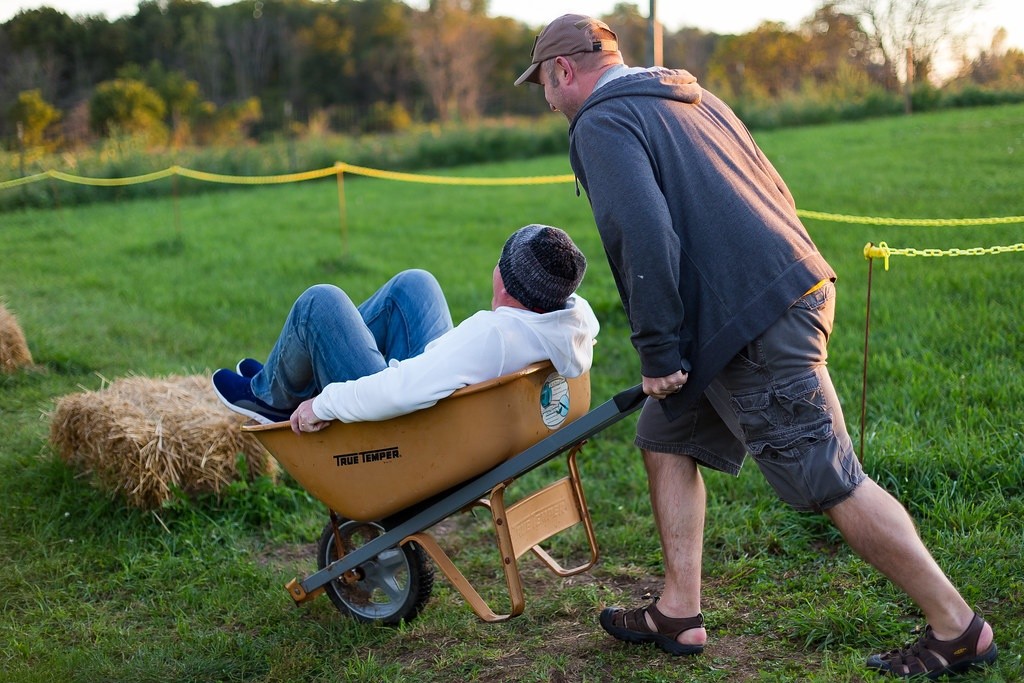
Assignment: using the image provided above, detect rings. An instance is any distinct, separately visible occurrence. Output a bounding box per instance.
[674,385,682,393]
[301,424,305,425]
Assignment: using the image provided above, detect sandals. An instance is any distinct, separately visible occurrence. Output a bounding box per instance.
[598,596,707,655]
[866,613,998,682]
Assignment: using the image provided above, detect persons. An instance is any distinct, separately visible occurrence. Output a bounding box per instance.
[212,223,600,435]
[514,14,997,683]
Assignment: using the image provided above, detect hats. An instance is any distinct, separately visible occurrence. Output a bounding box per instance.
[499,223,587,313]
[514,14,618,86]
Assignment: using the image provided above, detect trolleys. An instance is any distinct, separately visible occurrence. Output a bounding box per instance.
[241,338,694,631]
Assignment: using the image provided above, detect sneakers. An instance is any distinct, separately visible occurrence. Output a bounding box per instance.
[211,367,289,425]
[235,358,265,377]
